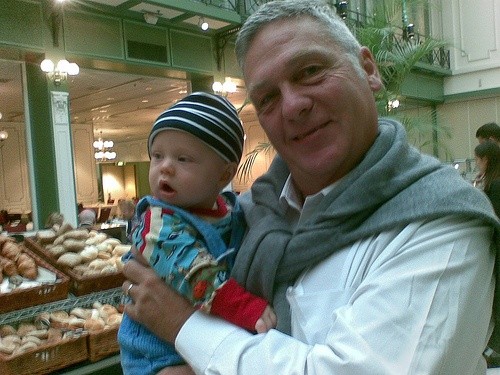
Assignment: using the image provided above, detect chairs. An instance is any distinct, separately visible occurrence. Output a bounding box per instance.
[0,198,140,233]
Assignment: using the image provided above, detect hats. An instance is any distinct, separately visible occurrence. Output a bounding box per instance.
[146,92,244,164]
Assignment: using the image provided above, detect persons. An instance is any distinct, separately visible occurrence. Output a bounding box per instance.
[121,1,496,374]
[474,144,500,336]
[116,91,277,373]
[476,122,499,143]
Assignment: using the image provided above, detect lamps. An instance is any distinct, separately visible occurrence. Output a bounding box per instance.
[40,59,80,86]
[338,1,348,20]
[406,24,415,40]
[92,137,116,164]
[212,80,237,98]
[201,17,209,31]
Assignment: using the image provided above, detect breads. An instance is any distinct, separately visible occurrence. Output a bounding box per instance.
[0,300,125,362]
[0,233,37,284]
[33,223,131,279]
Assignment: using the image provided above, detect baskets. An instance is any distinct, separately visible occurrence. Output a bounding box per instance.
[0,240,70,314]
[0,320,88,375]
[24,235,126,296]
[46,302,120,362]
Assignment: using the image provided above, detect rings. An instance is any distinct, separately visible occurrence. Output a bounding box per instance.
[125,284,137,297]
